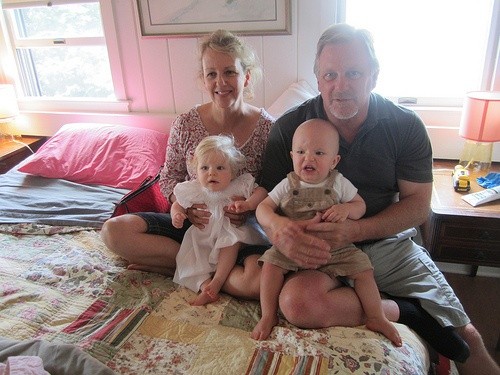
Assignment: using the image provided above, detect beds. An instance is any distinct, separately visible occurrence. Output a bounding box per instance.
[1,110,433,375]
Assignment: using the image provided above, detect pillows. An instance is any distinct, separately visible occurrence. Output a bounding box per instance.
[18,122,168,190]
[265,75,320,120]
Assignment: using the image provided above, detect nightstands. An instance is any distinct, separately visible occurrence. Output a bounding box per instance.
[0,136,45,174]
[420,161,500,268]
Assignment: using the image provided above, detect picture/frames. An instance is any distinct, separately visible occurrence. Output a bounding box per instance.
[136,0,293,39]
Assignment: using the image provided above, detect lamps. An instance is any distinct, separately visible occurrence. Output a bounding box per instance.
[460,92,500,177]
[0,82,19,125]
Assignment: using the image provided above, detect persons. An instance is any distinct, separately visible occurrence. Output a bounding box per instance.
[100,28,276,298]
[170,135,268,306]
[250,118,403,347]
[260,24,500,375]
